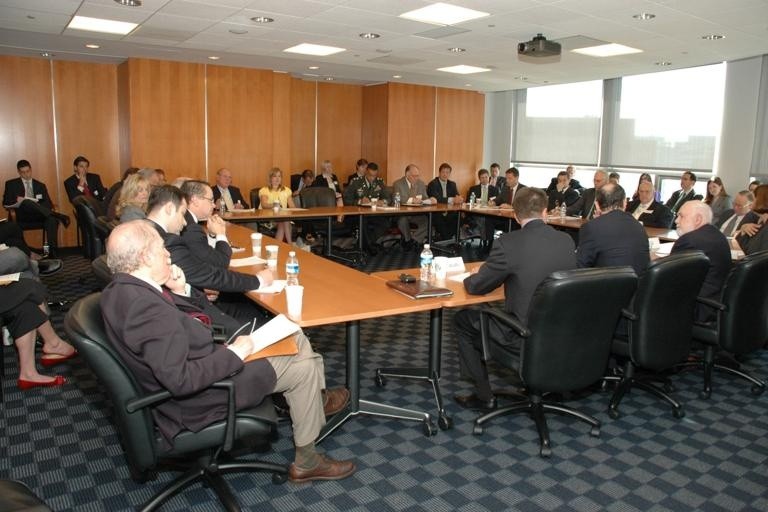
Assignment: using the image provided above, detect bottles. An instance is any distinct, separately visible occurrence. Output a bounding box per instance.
[285,251,299,287]
[394,192,400,210]
[220,194,225,216]
[419,244,433,282]
[560,201,566,218]
[469,191,476,208]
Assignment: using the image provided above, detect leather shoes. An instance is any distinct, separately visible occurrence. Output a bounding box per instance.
[290,457,358,484]
[321,386,351,415]
[62,215,71,229]
[452,393,499,416]
[41,346,79,366]
[36,257,63,277]
[18,374,68,390]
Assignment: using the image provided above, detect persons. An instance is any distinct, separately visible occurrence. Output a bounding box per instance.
[181,181,233,270]
[0,277,80,390]
[705,177,733,223]
[64,155,109,205]
[487,167,526,231]
[211,167,249,211]
[736,184,768,255]
[0,220,43,261]
[625,182,673,228]
[119,173,151,222]
[427,162,464,241]
[102,167,141,214]
[716,191,759,249]
[107,167,160,231]
[292,170,320,242]
[651,200,732,375]
[345,159,369,186]
[550,170,608,220]
[547,166,582,197]
[99,219,358,483]
[145,184,274,345]
[577,183,650,397]
[258,168,296,244]
[3,159,70,259]
[488,162,506,191]
[633,173,662,203]
[172,176,193,189]
[547,172,581,241]
[156,168,168,186]
[452,187,577,415]
[747,179,763,193]
[316,160,342,207]
[392,164,438,255]
[466,168,499,245]
[343,162,393,258]
[665,171,697,229]
[0,242,63,278]
[606,172,619,184]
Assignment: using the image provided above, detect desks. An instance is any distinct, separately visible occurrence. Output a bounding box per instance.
[371,242,744,432]
[461,202,678,252]
[214,194,468,265]
[203,221,443,446]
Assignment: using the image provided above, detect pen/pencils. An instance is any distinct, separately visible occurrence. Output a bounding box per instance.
[263,263,267,270]
[231,245,240,249]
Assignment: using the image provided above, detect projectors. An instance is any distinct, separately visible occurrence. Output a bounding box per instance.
[517,34,562,65]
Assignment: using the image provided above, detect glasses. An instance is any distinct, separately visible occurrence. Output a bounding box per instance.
[202,196,216,202]
[732,202,752,209]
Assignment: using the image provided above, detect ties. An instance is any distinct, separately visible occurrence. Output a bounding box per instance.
[25,181,33,198]
[83,181,91,196]
[223,191,232,210]
[508,189,513,204]
[483,186,487,201]
[673,192,686,210]
[410,183,415,192]
[162,289,212,328]
[723,215,738,237]
[368,183,372,190]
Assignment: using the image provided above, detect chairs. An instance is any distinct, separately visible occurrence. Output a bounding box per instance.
[72,194,110,256]
[90,256,114,287]
[679,249,768,401]
[249,188,260,206]
[63,290,289,512]
[299,186,356,264]
[602,248,710,420]
[472,264,640,458]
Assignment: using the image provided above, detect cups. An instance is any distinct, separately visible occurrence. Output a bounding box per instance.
[435,257,447,279]
[371,198,377,211]
[283,285,307,319]
[272,200,280,216]
[265,244,279,270]
[250,233,263,256]
